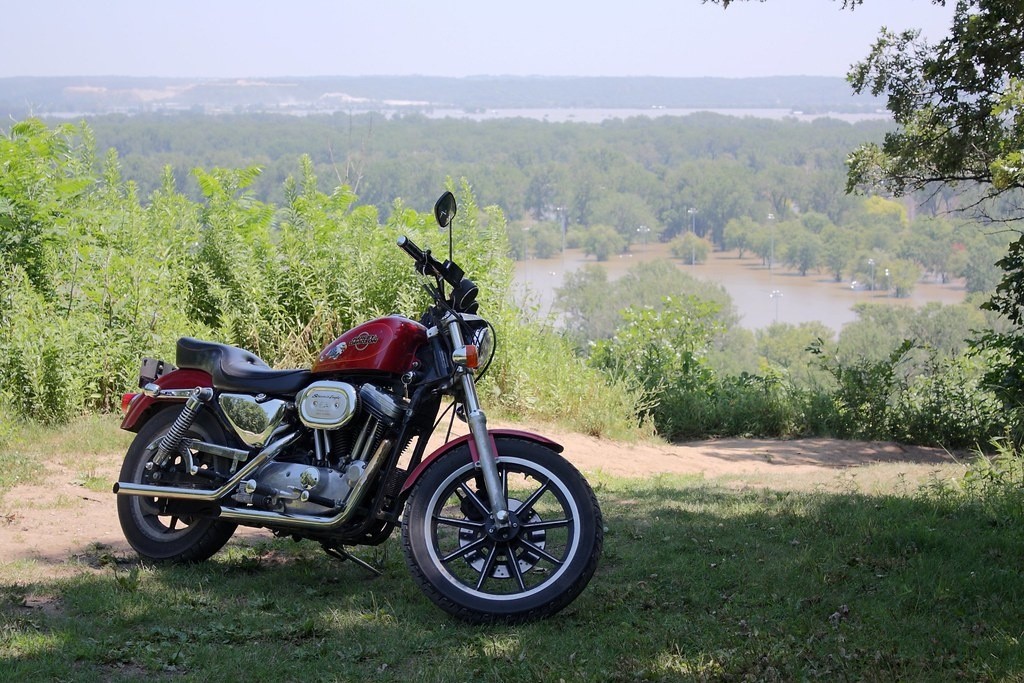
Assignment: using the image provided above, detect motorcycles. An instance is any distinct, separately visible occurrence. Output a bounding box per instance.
[110,192,607,623]
